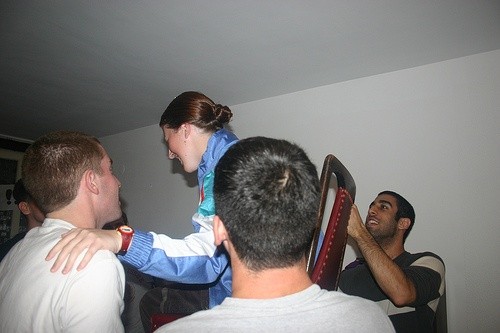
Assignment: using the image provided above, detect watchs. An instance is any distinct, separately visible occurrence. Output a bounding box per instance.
[116,224,136,256]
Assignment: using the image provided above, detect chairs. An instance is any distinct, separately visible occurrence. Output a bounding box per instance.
[151,154,355,333]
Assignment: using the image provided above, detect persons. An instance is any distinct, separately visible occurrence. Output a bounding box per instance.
[1,129,131,332]
[150,134,397,331]
[335,189,447,332]
[45,90,243,309]
[1,176,46,259]
